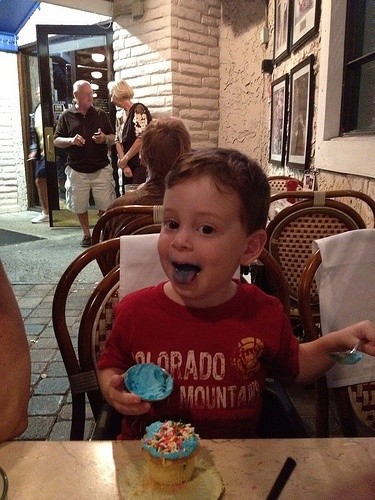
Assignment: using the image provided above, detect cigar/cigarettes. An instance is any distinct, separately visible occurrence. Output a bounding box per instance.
[27,157,32,161]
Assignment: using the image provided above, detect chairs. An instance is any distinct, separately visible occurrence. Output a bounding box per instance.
[52,176,375,442]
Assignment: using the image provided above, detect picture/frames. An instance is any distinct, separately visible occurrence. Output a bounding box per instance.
[290,0,321,51]
[266,74,290,167]
[287,54,316,172]
[271,0,291,65]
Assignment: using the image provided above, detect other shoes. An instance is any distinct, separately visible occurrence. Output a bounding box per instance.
[80,235,92,246]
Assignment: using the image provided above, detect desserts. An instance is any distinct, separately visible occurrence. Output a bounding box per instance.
[140,419,202,486]
[124,362,174,401]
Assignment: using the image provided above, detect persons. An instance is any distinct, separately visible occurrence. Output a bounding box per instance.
[107,116,191,210]
[97,148,375,440]
[52,80,115,246]
[0,257,31,445]
[27,86,49,223]
[107,79,152,195]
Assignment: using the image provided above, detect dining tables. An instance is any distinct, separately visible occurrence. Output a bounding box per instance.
[0,438,375,500]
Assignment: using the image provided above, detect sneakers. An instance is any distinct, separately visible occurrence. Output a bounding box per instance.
[31,211,54,223]
